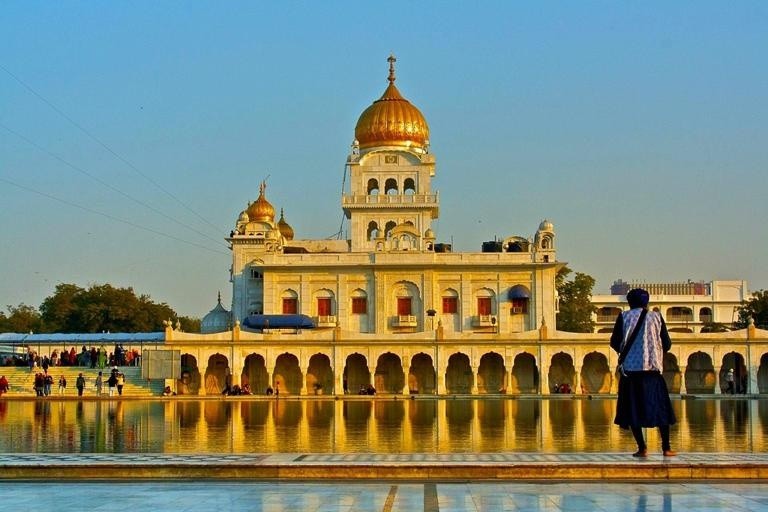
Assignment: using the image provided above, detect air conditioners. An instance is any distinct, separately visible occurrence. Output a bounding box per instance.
[513,307,523,313]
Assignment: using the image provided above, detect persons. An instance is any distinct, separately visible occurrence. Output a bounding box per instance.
[723,369,735,394]
[738,374,748,394]
[162,386,173,397]
[632,485,672,512]
[358,383,377,396]
[608,289,678,456]
[222,372,254,396]
[344,374,352,394]
[554,383,572,394]
[266,381,280,398]
[0,345,142,397]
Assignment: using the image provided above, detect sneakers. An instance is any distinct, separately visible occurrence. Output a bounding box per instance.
[662,450,677,456]
[632,448,648,457]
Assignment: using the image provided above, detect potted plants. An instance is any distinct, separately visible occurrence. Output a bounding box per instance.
[313,381,324,395]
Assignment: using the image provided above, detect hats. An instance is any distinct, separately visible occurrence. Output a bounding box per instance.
[627,289,649,305]
